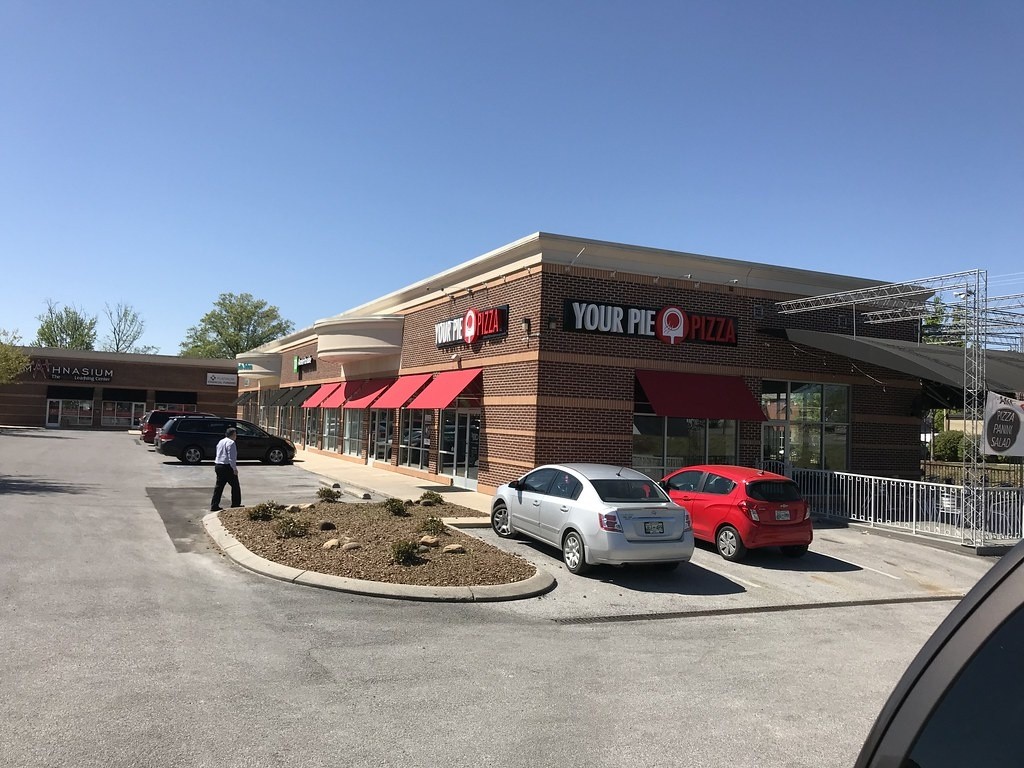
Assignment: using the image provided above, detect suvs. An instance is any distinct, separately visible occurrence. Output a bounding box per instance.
[157,415,297,468]
[139,410,216,444]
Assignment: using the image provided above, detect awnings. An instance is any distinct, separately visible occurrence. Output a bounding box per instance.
[320,381,364,408]
[371,373,433,408]
[406,368,482,410]
[274,387,304,406]
[301,383,340,408]
[343,378,393,409]
[239,392,258,405]
[265,389,289,405]
[233,393,249,404]
[287,384,321,406]
[635,370,768,421]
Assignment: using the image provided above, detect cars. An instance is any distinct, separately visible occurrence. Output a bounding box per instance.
[659,463,821,548]
[492,460,695,572]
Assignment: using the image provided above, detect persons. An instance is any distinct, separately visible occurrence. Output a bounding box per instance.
[209,428,244,511]
